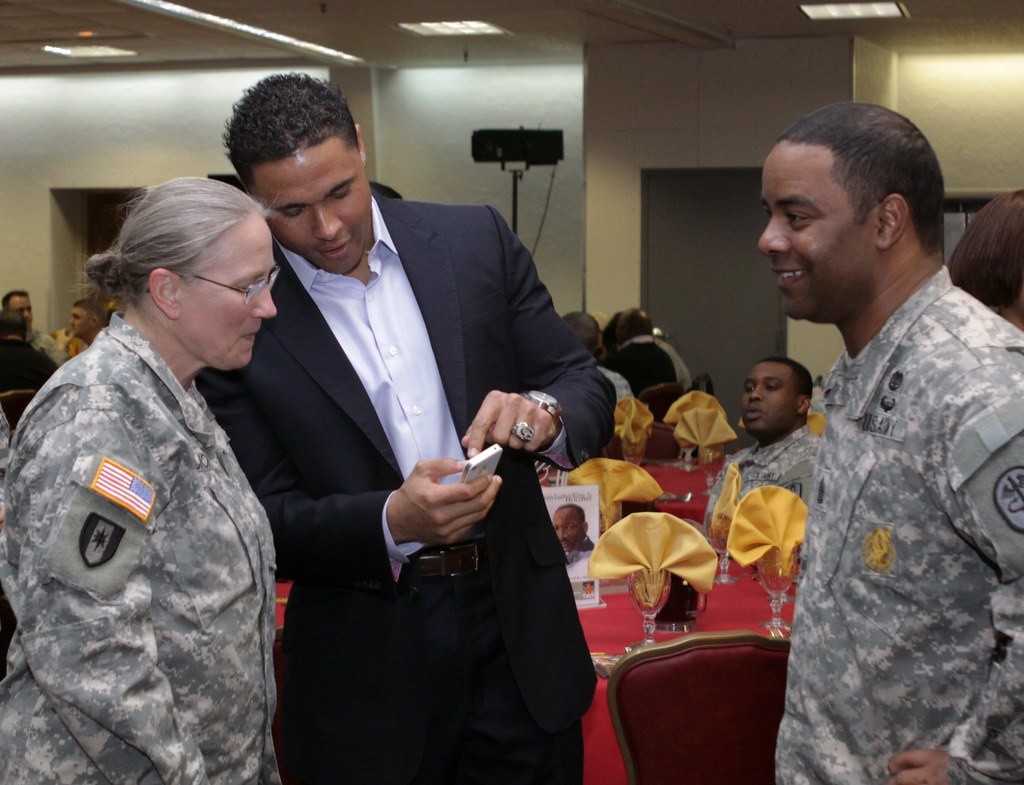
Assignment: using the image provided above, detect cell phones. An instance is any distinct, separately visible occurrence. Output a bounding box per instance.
[459,443,503,484]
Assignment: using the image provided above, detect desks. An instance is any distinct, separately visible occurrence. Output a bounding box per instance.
[537,453,801,785]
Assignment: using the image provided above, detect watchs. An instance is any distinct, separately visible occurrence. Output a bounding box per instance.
[520,390,562,431]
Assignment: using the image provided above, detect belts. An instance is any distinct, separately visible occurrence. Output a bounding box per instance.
[418,540,490,578]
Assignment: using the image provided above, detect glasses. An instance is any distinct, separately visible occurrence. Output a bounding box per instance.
[179,264,280,304]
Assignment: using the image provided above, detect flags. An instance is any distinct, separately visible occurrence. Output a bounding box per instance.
[93,458,156,522]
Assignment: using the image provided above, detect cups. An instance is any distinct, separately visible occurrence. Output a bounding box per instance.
[622,443,646,467]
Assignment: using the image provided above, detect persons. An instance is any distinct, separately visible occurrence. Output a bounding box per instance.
[705,355,822,557]
[0,176,282,785]
[558,307,692,459]
[758,103,1024,785]
[552,502,595,557]
[194,74,618,785]
[0,290,126,534]
[947,187,1024,333]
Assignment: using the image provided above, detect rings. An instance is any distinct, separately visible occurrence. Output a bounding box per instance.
[511,422,535,442]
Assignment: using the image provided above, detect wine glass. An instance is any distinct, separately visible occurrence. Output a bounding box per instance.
[697,444,724,496]
[678,439,698,471]
[625,566,672,653]
[757,545,797,630]
[705,512,740,584]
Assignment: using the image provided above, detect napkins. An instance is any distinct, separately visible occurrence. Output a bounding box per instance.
[587,510,719,604]
[568,388,827,542]
[723,484,810,577]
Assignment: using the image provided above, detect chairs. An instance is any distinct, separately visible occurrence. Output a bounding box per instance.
[0,389,40,436]
[606,629,791,784]
